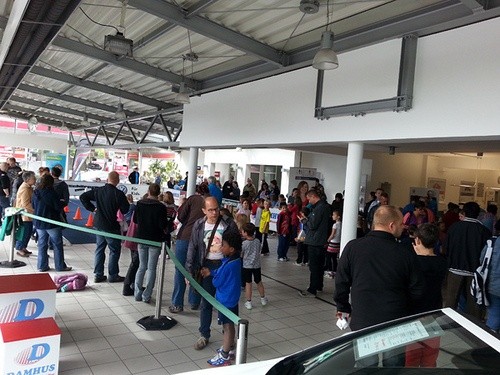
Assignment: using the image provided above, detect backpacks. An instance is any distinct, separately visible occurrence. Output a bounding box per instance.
[470,265,490,306]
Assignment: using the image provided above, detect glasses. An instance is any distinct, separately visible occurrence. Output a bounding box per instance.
[418,207,425,210]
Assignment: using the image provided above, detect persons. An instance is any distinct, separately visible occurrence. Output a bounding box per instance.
[296,188,333,298]
[333,205,413,367]
[240,223,270,309]
[79,170,130,284]
[159,191,178,259]
[360,189,500,255]
[332,193,344,208]
[0,157,69,250]
[236,213,248,230]
[219,207,231,218]
[128,166,140,184]
[296,180,310,207]
[169,183,211,313]
[442,201,489,321]
[132,182,169,302]
[275,187,311,266]
[32,173,72,272]
[14,171,38,258]
[403,224,452,368]
[323,209,342,279]
[184,195,238,360]
[479,220,500,334]
[166,171,240,201]
[200,230,243,367]
[240,178,280,207]
[122,193,149,296]
[235,198,272,256]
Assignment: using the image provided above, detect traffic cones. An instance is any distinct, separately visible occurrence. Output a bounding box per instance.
[64,205,69,213]
[73,207,82,220]
[85,212,94,227]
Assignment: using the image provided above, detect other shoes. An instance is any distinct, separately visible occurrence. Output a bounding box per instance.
[169,305,183,312]
[279,257,287,261]
[24,250,32,254]
[261,253,269,256]
[61,267,72,271]
[191,305,198,310]
[17,252,29,257]
[40,266,50,271]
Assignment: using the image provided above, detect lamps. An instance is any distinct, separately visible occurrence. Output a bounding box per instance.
[175,60,191,104]
[81,105,90,127]
[115,85,127,119]
[311,0,338,71]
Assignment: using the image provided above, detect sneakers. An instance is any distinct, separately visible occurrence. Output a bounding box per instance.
[292,261,309,266]
[109,275,125,282]
[323,272,332,279]
[299,290,315,297]
[307,287,322,292]
[95,276,107,282]
[216,345,234,359]
[207,351,230,367]
[244,301,252,309]
[332,272,335,279]
[261,298,266,305]
[193,337,208,350]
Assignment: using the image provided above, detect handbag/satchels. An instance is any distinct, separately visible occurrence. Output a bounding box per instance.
[193,267,203,294]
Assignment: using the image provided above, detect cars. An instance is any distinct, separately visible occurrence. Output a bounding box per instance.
[170,308,500,375]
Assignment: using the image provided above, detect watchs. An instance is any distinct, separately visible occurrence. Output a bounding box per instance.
[7,194,9,196]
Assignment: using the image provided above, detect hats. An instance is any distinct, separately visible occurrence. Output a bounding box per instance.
[280,202,286,206]
